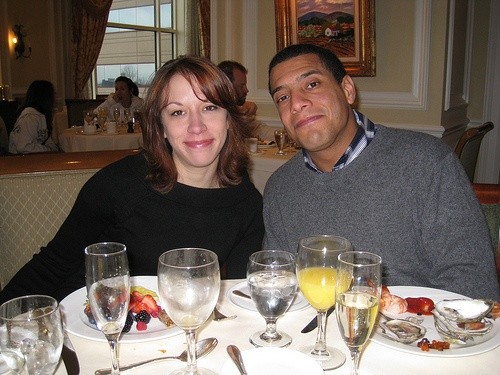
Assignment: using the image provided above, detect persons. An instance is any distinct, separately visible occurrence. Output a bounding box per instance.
[261,43,500,318]
[0,54,264,328]
[7,80,63,153]
[216,60,258,118]
[90,75,145,122]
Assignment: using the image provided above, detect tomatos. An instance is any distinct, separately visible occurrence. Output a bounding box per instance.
[404,297,436,315]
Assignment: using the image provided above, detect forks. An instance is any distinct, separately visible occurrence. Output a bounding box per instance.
[212,307,238,321]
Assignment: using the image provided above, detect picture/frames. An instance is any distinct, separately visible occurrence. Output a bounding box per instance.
[274,0,375,77]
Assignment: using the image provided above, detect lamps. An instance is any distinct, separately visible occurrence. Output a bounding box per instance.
[11,24,31,59]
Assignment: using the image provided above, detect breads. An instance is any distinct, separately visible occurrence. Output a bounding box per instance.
[368,280,408,314]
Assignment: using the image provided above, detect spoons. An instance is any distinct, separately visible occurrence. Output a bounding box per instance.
[94,337,218,375]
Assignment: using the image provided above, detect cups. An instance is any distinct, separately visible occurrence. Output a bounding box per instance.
[106,122,119,136]
[245,138,258,154]
[83,126,97,133]
[0,294,64,375]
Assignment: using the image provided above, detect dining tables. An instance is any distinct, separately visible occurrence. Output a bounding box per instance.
[0,280,500,375]
[245,144,300,196]
[59,126,143,151]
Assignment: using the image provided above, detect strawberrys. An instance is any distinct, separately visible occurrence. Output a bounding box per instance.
[84,287,162,319]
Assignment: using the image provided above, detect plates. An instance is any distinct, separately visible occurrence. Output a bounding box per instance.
[228,280,311,312]
[369,285,500,358]
[58,275,185,344]
[257,141,276,148]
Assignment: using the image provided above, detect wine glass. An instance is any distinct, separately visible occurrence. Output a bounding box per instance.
[157,248,220,375]
[293,234,354,370]
[246,249,298,349]
[82,107,144,136]
[84,242,131,375]
[333,252,383,375]
[274,130,296,156]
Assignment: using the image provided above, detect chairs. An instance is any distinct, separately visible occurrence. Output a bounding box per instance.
[455,121,494,182]
[0,150,139,293]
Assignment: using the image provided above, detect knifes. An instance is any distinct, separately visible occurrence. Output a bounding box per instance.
[43,308,80,375]
[300,304,335,333]
[61,332,80,375]
